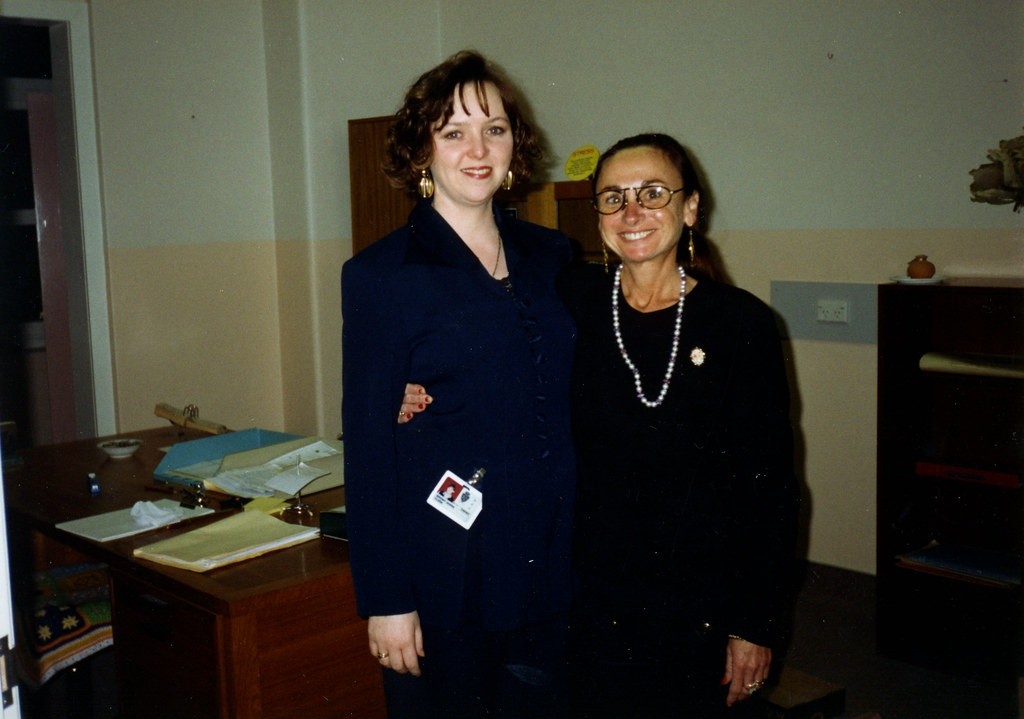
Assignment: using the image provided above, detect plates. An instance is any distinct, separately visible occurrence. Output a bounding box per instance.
[890,275,945,284]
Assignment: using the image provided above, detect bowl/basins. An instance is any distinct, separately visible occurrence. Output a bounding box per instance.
[97,439,144,459]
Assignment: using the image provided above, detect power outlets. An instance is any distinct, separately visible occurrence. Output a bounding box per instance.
[817,298,849,322]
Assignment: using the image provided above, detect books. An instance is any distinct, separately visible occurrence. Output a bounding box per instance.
[129,508,321,574]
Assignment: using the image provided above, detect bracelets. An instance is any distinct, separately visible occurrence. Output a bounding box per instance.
[726,634,739,640]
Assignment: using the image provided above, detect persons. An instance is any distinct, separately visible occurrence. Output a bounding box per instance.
[342,49,637,719]
[437,482,455,502]
[582,131,813,719]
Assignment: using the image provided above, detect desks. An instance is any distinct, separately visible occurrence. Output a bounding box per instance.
[2,425,388,719]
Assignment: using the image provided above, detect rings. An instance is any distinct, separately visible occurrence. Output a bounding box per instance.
[376,650,389,661]
[755,680,765,687]
[745,681,755,692]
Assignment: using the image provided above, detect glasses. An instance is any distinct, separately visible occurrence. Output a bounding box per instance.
[590,186,684,215]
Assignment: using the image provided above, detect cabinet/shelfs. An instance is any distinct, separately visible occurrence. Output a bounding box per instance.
[346,113,416,256]
[495,178,622,267]
[874,276,1024,682]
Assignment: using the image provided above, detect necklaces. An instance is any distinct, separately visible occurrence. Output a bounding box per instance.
[612,257,689,405]
[491,232,502,280]
